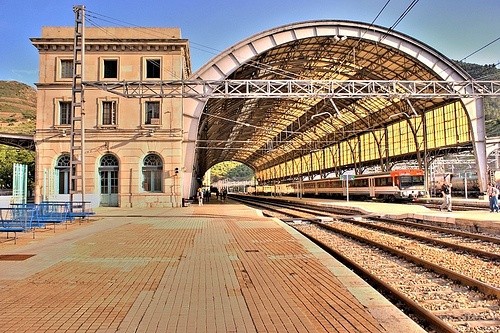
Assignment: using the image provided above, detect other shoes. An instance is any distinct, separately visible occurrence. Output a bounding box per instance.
[447,210,452,212]
[439,206,441,211]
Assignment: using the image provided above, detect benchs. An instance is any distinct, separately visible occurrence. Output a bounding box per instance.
[0,200,95,245]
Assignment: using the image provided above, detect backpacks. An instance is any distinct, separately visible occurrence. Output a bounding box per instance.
[443,183,451,193]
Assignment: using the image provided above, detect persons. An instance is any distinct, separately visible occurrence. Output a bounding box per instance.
[215,189,227,200]
[439,178,453,212]
[197,188,203,207]
[204,189,210,202]
[473,184,480,190]
[487,183,499,213]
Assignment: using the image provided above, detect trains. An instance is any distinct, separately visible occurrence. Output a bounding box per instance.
[248,169,426,203]
